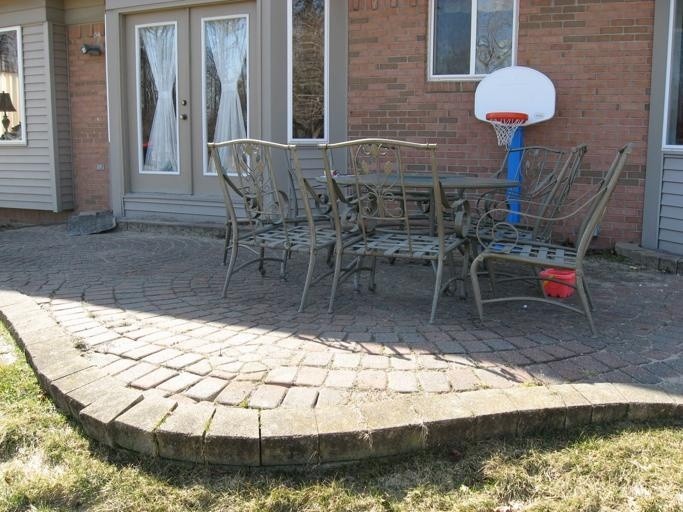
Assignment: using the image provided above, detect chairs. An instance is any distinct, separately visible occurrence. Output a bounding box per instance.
[437,141,637,339]
[315,135,473,323]
[206,136,364,314]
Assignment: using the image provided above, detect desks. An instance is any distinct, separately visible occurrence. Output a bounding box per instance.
[317,175,520,298]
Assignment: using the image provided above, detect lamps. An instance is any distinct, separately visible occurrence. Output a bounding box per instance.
[0,91,17,141]
